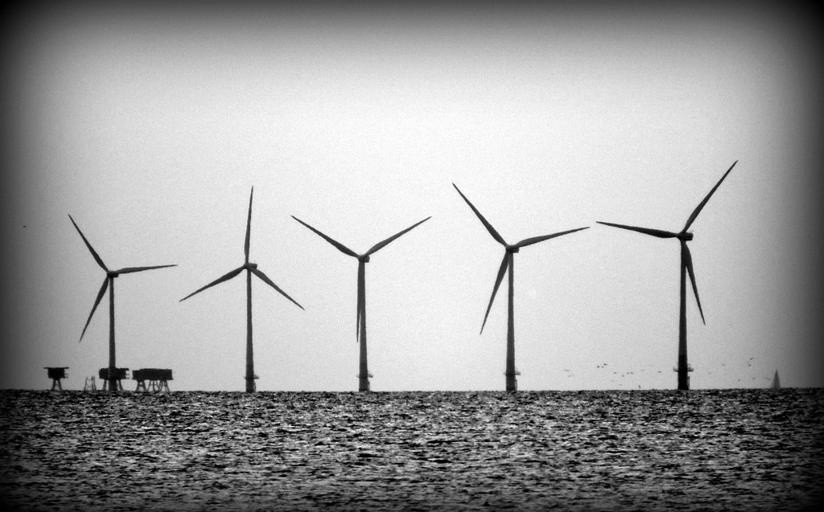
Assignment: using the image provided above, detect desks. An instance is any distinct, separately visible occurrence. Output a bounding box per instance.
[132,369,173,393]
[99,368,129,391]
[44,367,69,390]
